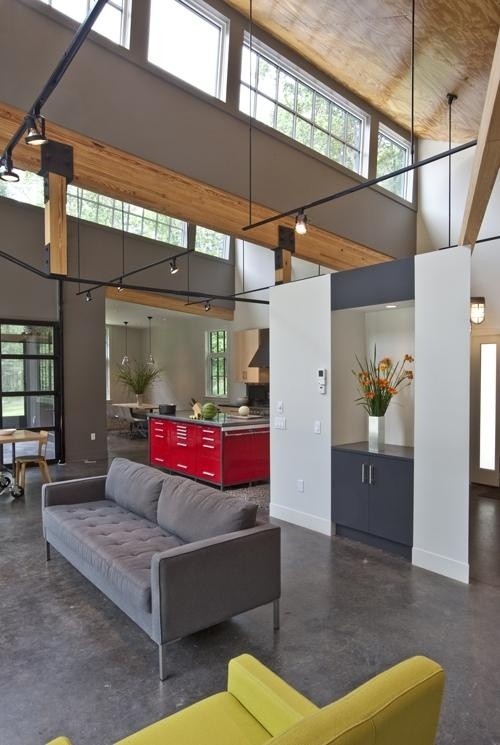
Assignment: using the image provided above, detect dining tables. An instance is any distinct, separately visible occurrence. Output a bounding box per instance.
[112,359,165,406]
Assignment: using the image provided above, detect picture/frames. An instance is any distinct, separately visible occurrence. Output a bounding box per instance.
[0,425,45,496]
[113,402,160,432]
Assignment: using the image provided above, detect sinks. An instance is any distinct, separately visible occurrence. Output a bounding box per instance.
[39,454,288,683]
[44,644,447,745]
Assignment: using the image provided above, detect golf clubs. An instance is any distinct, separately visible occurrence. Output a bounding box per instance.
[202,403,217,419]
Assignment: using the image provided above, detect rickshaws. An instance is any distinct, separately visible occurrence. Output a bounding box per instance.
[239,406,250,416]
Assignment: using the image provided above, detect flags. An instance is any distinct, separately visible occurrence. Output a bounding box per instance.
[366,414,387,454]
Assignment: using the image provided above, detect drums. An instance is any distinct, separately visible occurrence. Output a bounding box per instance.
[188,398,197,407]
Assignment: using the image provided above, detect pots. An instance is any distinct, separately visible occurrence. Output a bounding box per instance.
[159,404,176,414]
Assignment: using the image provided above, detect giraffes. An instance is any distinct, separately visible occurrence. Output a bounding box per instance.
[241,401,269,416]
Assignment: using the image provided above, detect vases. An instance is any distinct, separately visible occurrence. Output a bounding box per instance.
[348,341,416,415]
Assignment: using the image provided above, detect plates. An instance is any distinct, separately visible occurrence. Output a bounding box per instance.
[197,422,270,491]
[150,416,171,474]
[331,440,414,563]
[169,421,198,482]
[221,405,241,416]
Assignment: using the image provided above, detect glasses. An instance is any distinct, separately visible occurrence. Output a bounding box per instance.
[1,1,106,184]
[469,295,488,326]
[120,318,131,370]
[74,189,197,304]
[145,309,157,367]
[241,0,477,234]
[183,223,276,314]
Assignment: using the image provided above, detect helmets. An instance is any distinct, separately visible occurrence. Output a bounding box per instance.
[0,428,17,435]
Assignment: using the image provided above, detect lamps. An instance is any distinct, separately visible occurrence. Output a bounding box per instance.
[16,429,52,494]
[105,402,148,440]
